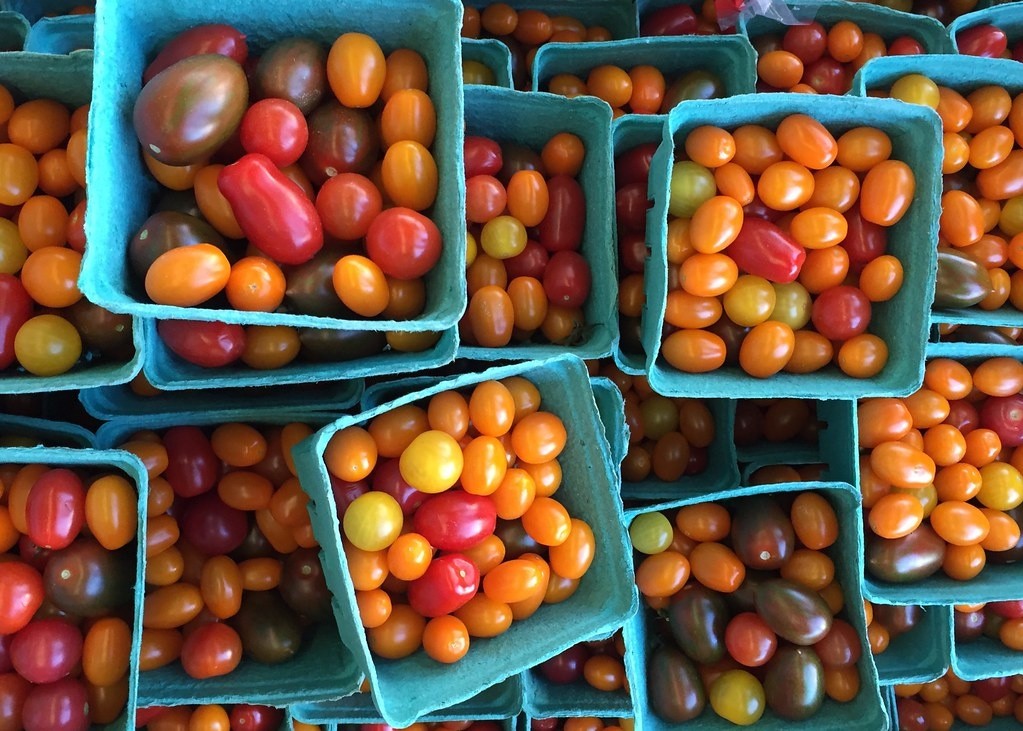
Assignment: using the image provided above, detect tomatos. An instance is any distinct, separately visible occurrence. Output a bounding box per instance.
[0,0,1023,731]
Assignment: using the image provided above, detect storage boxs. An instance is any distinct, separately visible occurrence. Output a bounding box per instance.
[0,0,1023,731]
